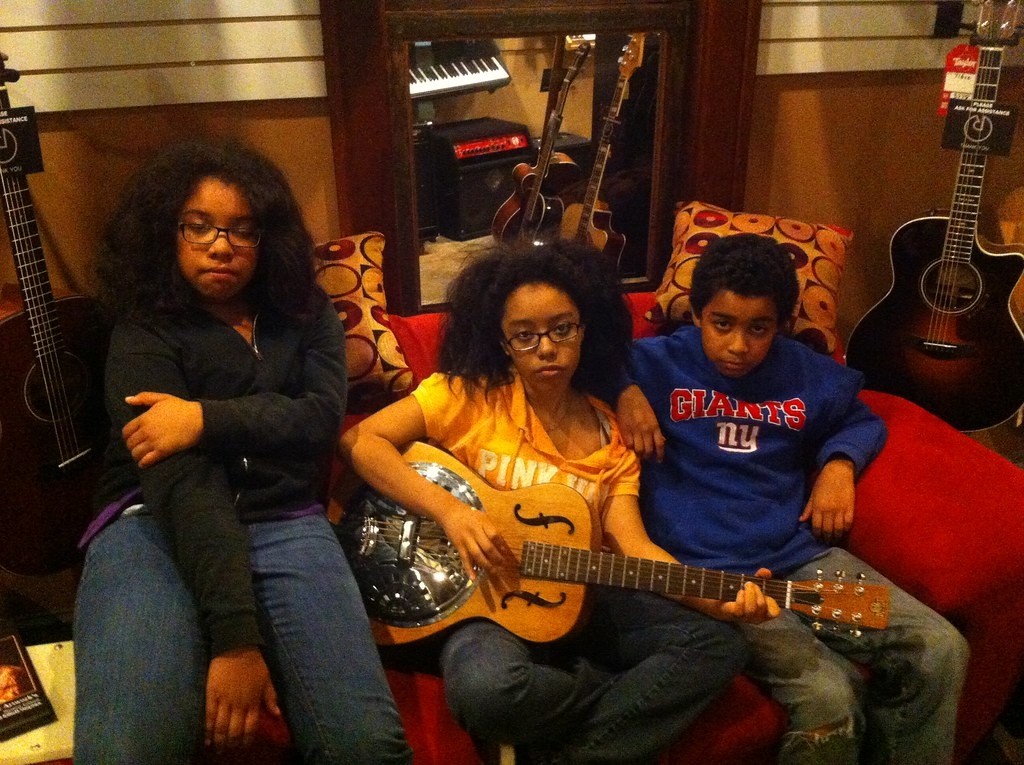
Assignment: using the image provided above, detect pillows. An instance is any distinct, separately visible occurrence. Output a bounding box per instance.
[307,229,416,394]
[646,200,855,370]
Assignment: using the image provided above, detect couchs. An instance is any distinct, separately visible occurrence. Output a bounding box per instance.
[257,293,1024,765]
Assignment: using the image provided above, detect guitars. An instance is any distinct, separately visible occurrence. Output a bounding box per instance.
[559,31,652,277]
[337,435,892,638]
[841,0,1024,437]
[490,34,592,247]
[0,50,121,578]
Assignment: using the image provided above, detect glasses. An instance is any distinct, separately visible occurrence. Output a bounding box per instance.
[177,217,266,248]
[503,318,586,351]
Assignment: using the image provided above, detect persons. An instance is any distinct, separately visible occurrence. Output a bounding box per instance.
[341,239,780,765]
[618,233,968,765]
[73,138,415,765]
